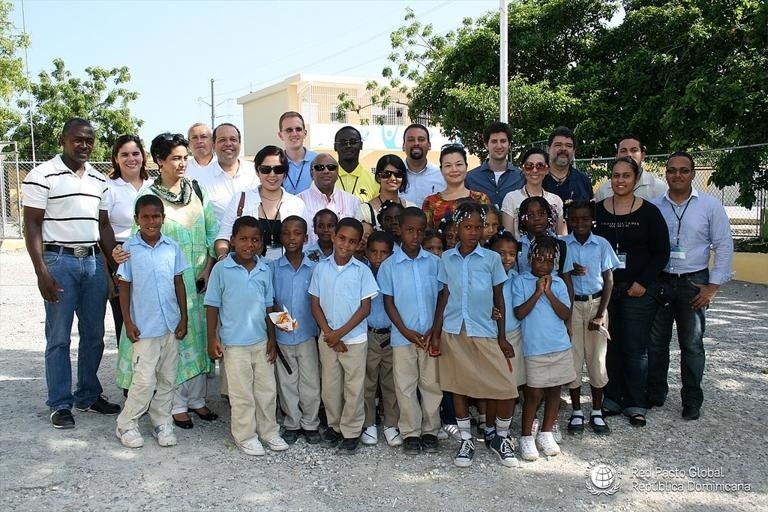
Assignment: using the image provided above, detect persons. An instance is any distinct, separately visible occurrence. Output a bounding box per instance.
[21,106,736,468]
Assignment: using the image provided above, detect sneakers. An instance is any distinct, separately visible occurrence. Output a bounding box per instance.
[52,390,698,466]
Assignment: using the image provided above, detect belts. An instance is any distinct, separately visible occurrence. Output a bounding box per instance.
[44,244,101,257]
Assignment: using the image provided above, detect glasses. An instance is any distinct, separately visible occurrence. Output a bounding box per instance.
[258,165,286,174]
[313,164,338,171]
[441,144,463,151]
[522,163,548,172]
[381,170,404,179]
[281,127,303,134]
[335,138,360,146]
[165,133,183,142]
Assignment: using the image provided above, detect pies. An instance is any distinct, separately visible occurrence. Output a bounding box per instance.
[277,310,297,330]
